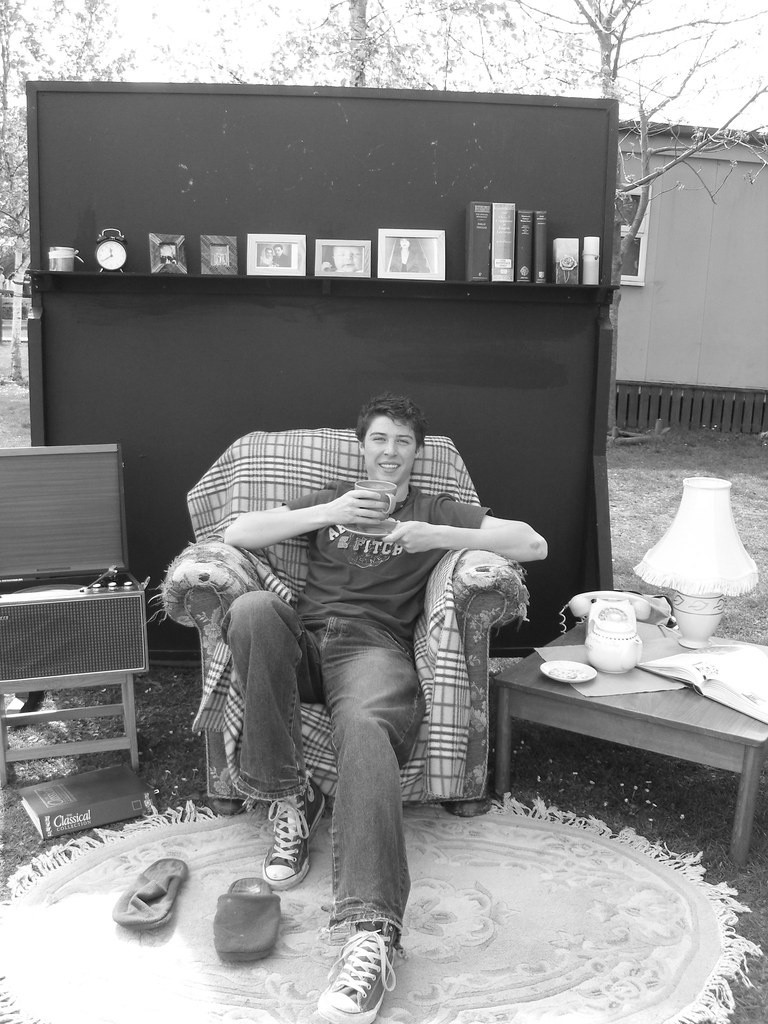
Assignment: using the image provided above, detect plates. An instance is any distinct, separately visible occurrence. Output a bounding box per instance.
[342,521,397,537]
[539,661,598,682]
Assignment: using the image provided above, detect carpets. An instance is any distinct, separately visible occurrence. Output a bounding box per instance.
[2,793,766,1024]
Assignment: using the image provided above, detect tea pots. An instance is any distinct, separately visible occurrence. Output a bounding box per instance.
[584,616,643,674]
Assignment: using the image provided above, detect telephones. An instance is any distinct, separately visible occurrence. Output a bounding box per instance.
[567,590,669,674]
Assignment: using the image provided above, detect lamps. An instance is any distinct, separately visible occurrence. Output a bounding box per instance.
[633,475,760,651]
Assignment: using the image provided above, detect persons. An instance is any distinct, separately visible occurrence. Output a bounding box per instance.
[323,246,362,273]
[260,244,289,267]
[222,394,548,1024]
[391,238,419,272]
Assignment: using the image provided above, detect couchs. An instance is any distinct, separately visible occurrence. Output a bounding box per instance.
[155,432,530,819]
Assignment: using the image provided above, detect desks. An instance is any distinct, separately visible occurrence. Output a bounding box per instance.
[490,619,768,868]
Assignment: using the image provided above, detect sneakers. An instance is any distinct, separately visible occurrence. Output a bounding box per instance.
[316,923,396,1024]
[263,779,325,889]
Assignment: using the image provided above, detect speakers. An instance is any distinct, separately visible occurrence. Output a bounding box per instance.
[0,584,149,684]
[0,442,128,584]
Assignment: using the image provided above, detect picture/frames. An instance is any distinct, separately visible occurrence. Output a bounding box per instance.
[246,232,307,277]
[314,239,371,278]
[198,234,240,275]
[377,228,447,281]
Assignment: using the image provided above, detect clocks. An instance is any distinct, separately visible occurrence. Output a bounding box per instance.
[94,229,129,274]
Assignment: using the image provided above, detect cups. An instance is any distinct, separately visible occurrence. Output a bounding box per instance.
[354,480,397,520]
[48,247,75,271]
[582,236,599,285]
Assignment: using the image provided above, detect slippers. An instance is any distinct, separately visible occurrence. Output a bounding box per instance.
[214,878,281,962]
[112,858,187,928]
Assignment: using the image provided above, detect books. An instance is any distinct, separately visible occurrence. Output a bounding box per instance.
[638,644,768,725]
[465,201,547,283]
